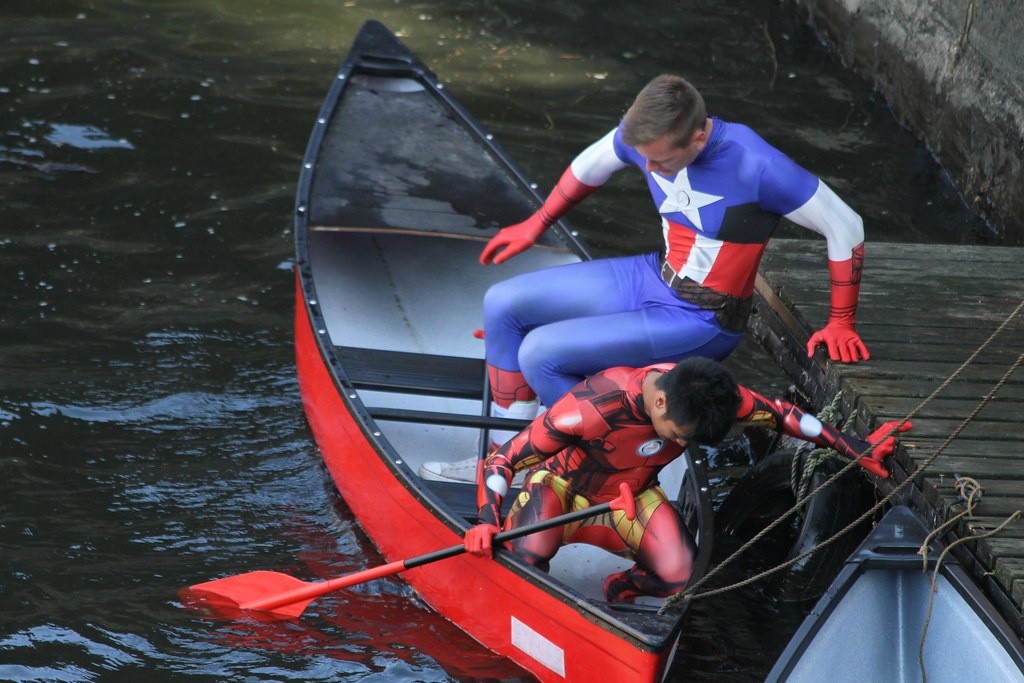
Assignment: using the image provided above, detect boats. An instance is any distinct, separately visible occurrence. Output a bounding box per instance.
[764,499,1024,683]
[294,19,1024,683]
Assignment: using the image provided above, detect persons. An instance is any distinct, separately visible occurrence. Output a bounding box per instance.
[464,356,913,609]
[415,73,866,487]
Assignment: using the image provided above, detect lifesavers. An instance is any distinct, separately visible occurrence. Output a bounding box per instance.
[712,448,871,606]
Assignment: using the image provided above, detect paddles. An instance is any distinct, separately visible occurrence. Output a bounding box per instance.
[188,482,636,618]
[474,329,492,484]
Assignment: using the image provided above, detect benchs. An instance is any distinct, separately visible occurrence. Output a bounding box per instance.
[334,344,493,413]
[425,479,519,524]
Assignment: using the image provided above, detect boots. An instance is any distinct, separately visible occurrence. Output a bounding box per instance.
[419,396,541,488]
